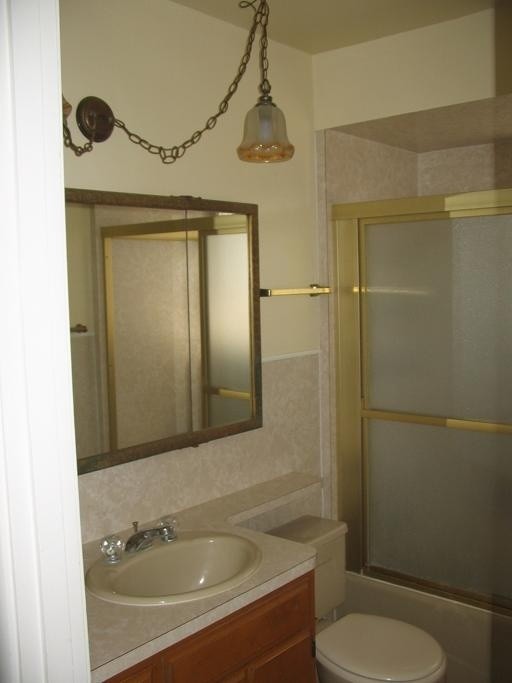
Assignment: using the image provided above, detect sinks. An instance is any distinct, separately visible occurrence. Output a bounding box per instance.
[86,530,265,615]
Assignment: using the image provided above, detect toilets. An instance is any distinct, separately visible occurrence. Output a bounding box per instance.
[264,514,447,683]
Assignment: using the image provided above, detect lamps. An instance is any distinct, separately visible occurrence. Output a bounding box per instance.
[236,2,297,166]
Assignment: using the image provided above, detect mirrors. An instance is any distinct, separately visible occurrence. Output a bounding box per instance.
[64,185,264,479]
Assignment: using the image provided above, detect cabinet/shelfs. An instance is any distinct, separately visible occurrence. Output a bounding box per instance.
[107,570,318,683]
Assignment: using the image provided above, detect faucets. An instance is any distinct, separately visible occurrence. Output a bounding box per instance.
[125,526,178,552]
